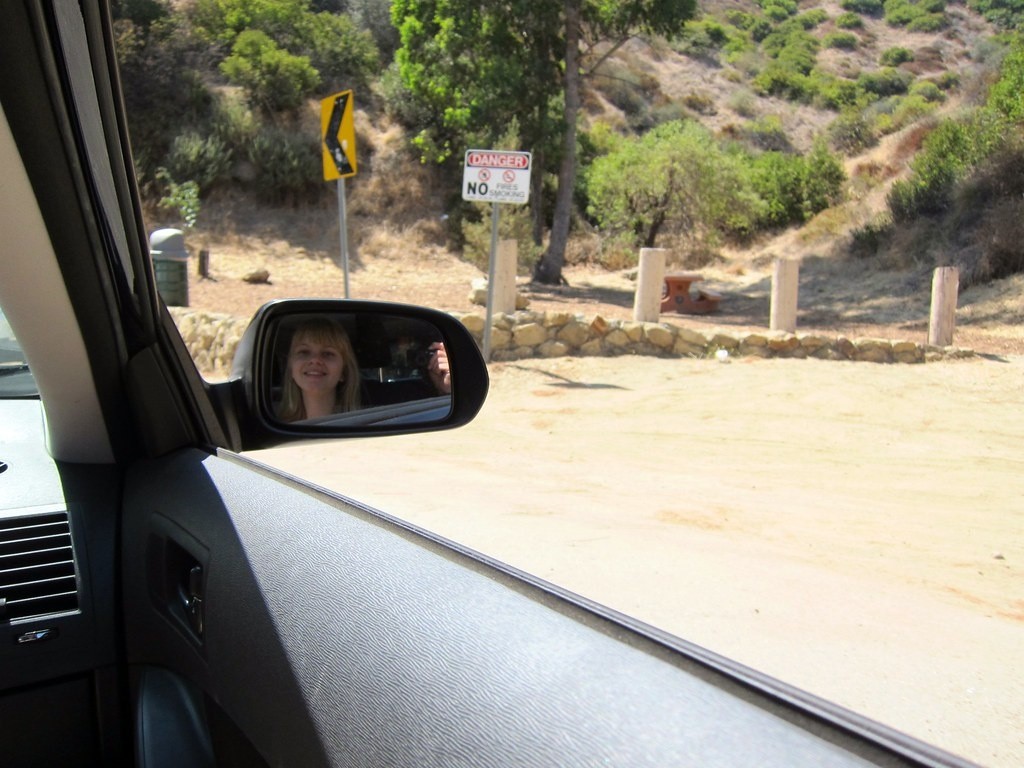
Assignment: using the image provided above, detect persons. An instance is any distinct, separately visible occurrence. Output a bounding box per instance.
[274,316,361,425]
[415,331,453,398]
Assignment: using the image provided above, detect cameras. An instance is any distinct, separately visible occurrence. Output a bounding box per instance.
[406,348,438,368]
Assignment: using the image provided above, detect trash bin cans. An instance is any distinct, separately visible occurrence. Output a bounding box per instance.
[150,228,190,306]
